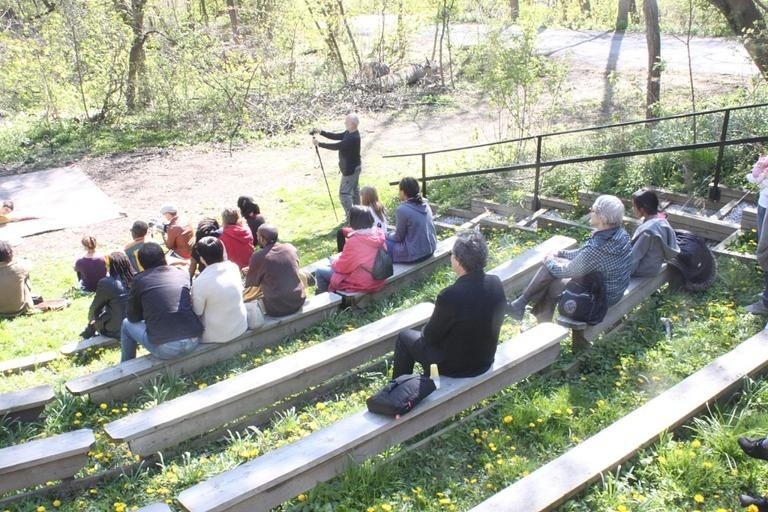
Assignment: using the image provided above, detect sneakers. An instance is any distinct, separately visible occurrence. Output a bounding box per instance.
[506,302,524,320]
[746,300,768,314]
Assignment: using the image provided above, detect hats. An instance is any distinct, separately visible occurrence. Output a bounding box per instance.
[161,205,176,215]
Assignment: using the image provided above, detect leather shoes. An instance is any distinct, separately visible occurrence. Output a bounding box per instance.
[740,495,768,512]
[738,437,768,459]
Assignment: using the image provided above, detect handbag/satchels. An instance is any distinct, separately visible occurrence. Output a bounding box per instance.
[366,374,436,416]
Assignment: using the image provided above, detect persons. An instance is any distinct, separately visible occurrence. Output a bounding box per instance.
[315,205,387,296]
[120,243,203,363]
[739,435,768,512]
[742,151,768,319]
[240,224,306,319]
[189,236,248,344]
[505,194,632,332]
[68,196,265,340]
[630,188,681,278]
[392,230,507,381]
[313,115,363,229]
[336,177,437,265]
[1,239,31,315]
[0,201,32,224]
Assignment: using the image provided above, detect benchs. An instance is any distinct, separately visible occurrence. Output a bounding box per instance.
[294,251,346,281]
[0,351,60,375]
[554,240,673,354]
[174,321,570,512]
[104,303,433,459]
[466,326,767,512]
[331,234,472,309]
[0,384,56,422]
[138,502,171,512]
[484,233,578,297]
[65,289,342,403]
[59,335,118,355]
[0,428,96,492]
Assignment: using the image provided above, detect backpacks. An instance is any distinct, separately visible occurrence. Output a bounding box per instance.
[361,245,393,280]
[558,271,607,325]
